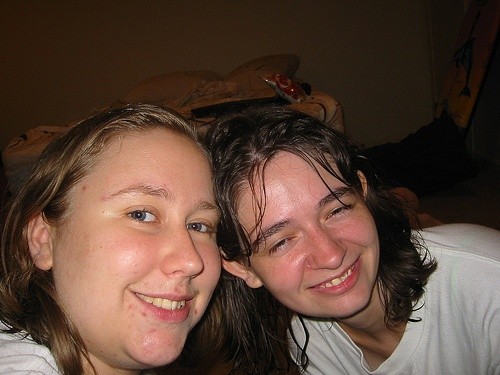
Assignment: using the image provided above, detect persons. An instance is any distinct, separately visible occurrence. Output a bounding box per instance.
[1,101,222,374]
[197,104,500,374]
